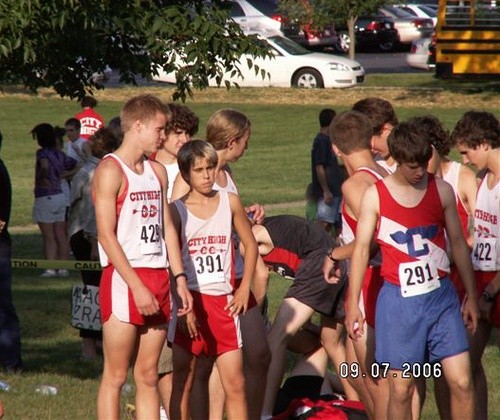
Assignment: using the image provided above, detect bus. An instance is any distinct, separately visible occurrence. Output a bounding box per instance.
[433,0,500,79]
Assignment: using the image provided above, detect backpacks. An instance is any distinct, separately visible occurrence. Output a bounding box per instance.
[268,393,370,420]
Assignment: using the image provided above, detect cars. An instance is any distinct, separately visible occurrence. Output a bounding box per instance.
[264,3,436,71]
[148,32,366,89]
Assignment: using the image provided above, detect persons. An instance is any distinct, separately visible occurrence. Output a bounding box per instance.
[0,93,500,420]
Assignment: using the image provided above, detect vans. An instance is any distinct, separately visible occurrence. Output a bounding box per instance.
[193,0,308,50]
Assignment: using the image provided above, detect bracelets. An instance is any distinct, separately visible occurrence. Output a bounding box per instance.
[174,273,188,282]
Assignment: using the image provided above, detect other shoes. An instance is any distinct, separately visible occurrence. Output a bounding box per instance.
[80,354,104,367]
[38,268,70,278]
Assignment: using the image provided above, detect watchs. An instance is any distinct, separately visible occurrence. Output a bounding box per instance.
[482,291,494,303]
[327,247,337,262]
